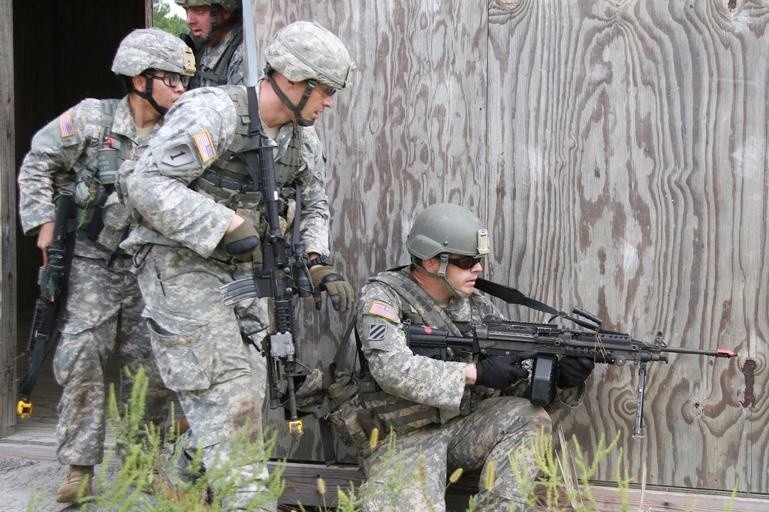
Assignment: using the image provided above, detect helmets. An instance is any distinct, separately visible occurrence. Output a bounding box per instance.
[264,20,355,89]
[175,0,242,12]
[406,203,491,261]
[111,25,198,79]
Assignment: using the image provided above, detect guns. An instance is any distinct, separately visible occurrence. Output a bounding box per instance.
[401,307,737,439]
[16,191,75,417]
[220,145,315,420]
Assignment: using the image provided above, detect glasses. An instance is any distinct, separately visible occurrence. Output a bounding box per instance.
[434,255,482,270]
[308,80,337,96]
[144,72,189,88]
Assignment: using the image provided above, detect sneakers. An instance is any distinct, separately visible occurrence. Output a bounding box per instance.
[56,460,95,504]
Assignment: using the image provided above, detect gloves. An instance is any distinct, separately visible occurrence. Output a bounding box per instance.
[309,264,354,312]
[475,351,540,390]
[223,220,263,275]
[556,354,594,389]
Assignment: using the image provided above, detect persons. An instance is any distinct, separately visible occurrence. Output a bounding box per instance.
[175,0,243,90]
[118,20,357,511]
[17,26,198,505]
[325,202,595,511]
